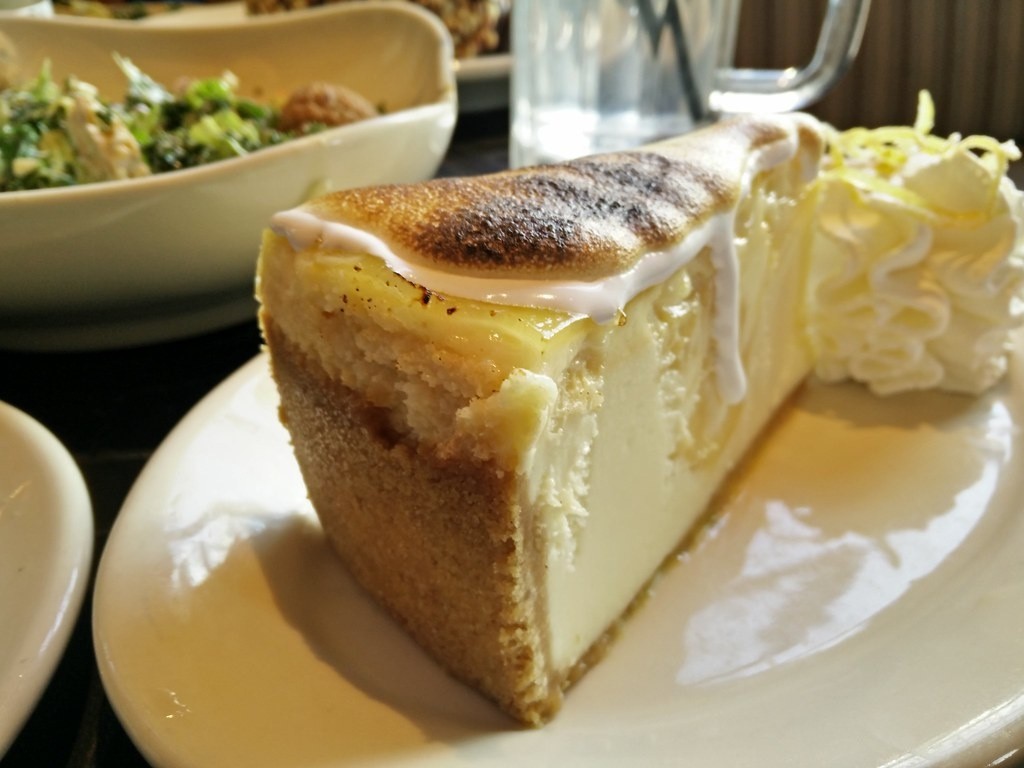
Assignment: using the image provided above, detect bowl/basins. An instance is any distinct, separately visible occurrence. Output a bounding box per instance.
[2,1,458,354]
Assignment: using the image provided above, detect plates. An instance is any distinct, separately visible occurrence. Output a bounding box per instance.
[0,403,94,763]
[95,342,1024,768]
[138,2,509,113]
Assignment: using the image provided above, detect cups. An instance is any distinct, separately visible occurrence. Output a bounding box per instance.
[508,0,868,169]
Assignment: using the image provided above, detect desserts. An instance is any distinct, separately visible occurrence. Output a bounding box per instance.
[251,90,1024,727]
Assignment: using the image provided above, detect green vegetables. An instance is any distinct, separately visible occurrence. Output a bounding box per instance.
[0,47,331,195]
